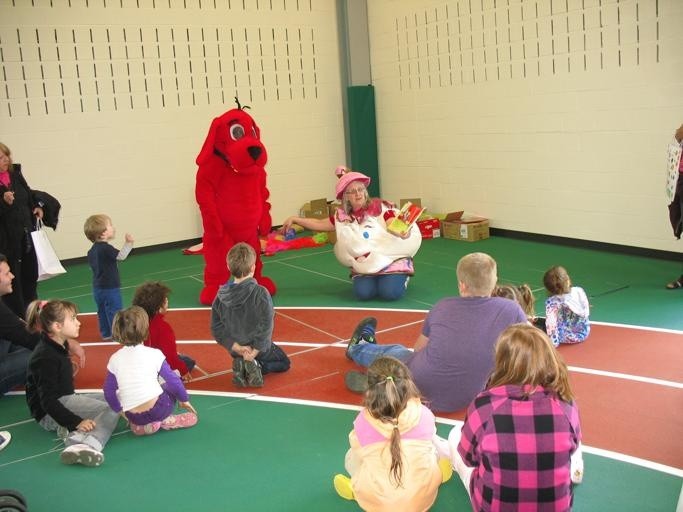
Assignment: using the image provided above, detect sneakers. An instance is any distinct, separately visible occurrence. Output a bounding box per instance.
[569,439,584,484]
[438,457,453,483]
[345,315,378,362]
[159,411,198,431]
[60,443,104,467]
[129,420,161,436]
[72,360,80,377]
[0,430,12,451]
[230,356,264,389]
[333,473,355,500]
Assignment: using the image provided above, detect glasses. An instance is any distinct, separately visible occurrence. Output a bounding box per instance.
[344,186,368,195]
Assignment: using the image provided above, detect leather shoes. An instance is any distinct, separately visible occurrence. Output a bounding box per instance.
[182,247,204,255]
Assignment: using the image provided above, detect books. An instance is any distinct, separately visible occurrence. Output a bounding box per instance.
[388,201,427,236]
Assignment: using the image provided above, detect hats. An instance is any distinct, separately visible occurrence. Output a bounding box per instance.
[335,166,371,202]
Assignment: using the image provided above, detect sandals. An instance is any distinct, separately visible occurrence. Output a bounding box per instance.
[666,274,683,289]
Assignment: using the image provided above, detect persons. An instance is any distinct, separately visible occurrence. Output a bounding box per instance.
[282,167,422,302]
[210,241,290,388]
[492,283,534,316]
[449,324,584,512]
[131,282,196,384]
[0,253,86,396]
[0,142,44,320]
[531,265,590,350]
[24,299,122,467]
[346,253,528,413]
[665,124,683,289]
[103,306,198,436]
[84,215,134,341]
[332,357,453,512]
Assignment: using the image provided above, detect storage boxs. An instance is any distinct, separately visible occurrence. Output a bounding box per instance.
[397,198,489,242]
[296,195,340,246]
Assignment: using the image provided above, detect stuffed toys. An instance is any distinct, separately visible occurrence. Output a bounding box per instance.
[197,97,277,305]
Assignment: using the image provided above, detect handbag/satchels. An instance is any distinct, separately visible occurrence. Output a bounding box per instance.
[664,131,683,206]
[29,216,69,283]
